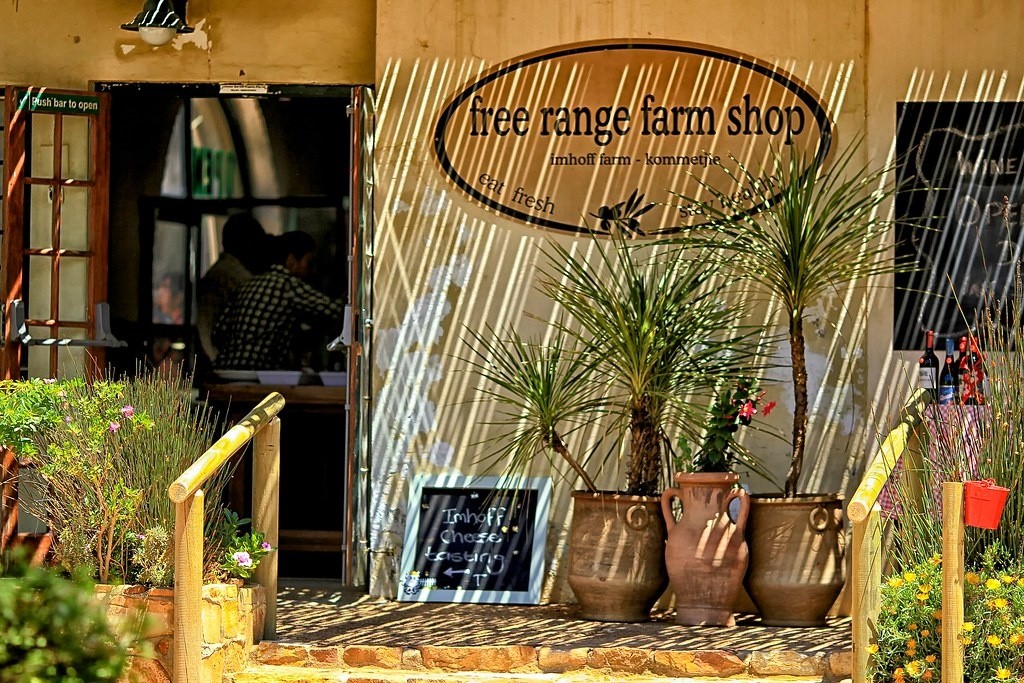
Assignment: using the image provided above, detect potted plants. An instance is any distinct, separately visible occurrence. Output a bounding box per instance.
[450,188,669,622]
[631,116,960,628]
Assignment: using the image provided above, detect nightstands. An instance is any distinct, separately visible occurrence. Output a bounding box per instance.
[876,404,1013,526]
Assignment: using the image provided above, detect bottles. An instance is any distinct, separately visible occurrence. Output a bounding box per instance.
[919,331,939,404]
[954,337,968,405]
[939,336,958,405]
[964,337,983,405]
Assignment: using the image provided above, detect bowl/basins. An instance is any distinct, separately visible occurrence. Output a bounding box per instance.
[257,371,302,387]
[319,372,347,386]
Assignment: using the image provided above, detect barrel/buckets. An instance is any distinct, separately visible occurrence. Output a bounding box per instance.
[963,478,1010,530]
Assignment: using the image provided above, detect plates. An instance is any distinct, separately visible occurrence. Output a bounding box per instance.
[215,370,257,380]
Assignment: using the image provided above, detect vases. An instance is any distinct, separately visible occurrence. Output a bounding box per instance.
[661,472,750,624]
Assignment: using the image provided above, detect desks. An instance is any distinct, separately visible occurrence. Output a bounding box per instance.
[205,382,346,551]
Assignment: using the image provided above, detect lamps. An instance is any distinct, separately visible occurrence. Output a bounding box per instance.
[121,0,195,46]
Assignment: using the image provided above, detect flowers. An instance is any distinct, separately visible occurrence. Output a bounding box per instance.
[0,331,279,586]
[688,376,778,472]
[865,545,1024,683]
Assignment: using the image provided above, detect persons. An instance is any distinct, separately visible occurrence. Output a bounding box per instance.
[155,211,347,371]
